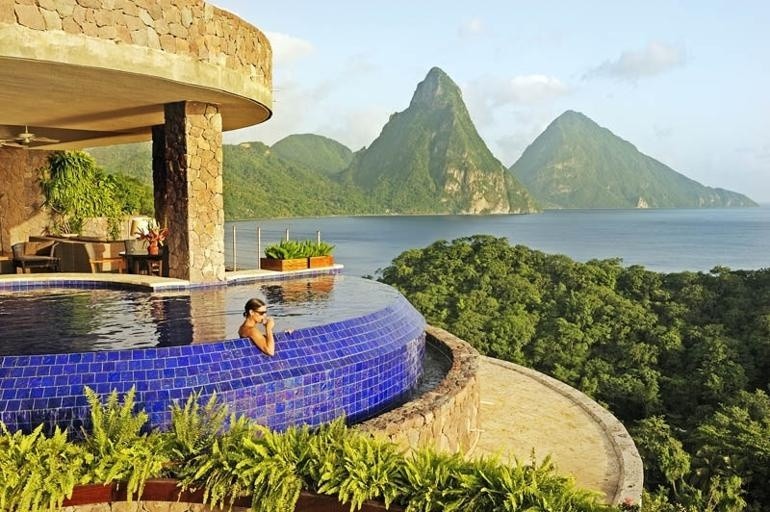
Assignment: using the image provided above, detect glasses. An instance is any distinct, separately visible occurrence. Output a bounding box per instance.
[253,309,267,316]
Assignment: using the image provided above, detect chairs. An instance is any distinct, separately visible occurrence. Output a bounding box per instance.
[13,233,138,276]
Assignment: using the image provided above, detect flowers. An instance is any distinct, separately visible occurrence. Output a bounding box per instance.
[136,219,169,245]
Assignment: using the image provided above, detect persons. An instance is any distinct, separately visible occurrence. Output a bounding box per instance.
[238,299,294,356]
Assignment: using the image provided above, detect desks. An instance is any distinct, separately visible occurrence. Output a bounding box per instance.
[118,249,164,276]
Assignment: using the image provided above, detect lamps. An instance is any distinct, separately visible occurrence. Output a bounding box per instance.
[130,217,148,238]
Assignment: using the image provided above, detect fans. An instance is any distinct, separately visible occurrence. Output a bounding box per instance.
[0,126,59,149]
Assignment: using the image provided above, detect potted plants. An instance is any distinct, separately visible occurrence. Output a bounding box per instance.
[302,241,334,270]
[261,240,309,272]
